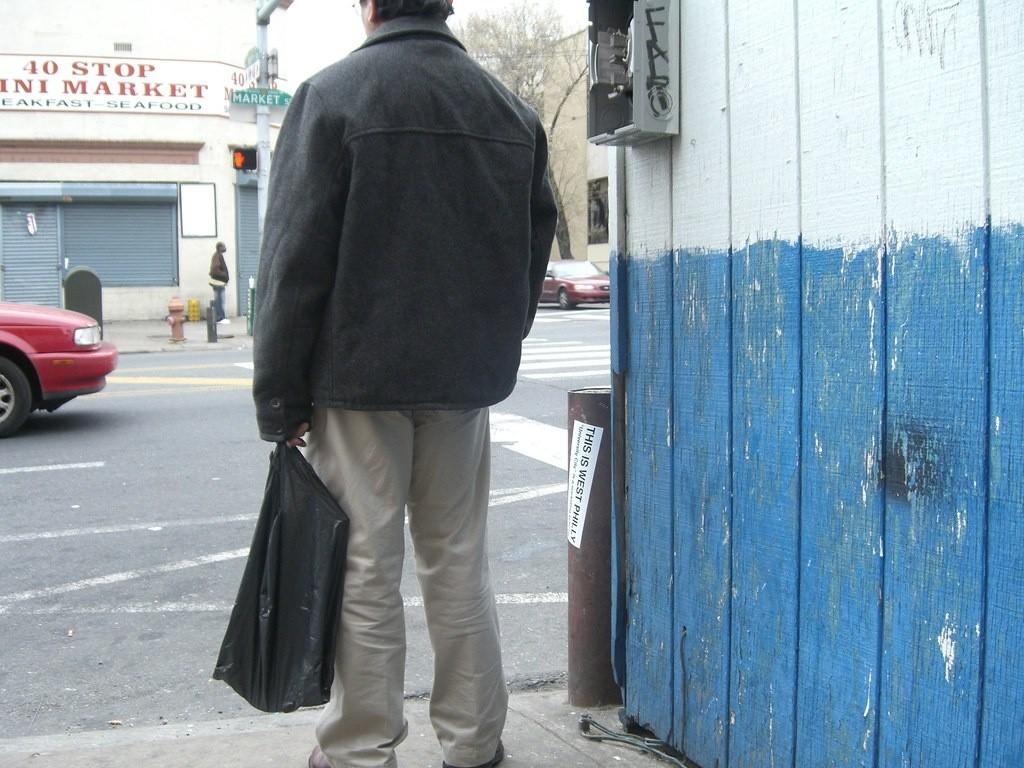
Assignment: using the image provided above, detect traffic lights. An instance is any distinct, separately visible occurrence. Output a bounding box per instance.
[233,148,259,172]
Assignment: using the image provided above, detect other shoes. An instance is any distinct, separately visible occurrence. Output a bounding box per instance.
[216,319,230,324]
[443,739,504,768]
[309,745,332,768]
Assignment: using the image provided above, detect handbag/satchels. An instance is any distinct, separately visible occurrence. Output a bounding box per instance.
[212,441,350,713]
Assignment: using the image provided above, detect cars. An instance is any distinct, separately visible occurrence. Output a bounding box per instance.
[535,259,610,310]
[0,299,119,441]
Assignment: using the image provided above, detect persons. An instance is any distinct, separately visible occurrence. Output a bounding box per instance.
[250,0,557,768]
[209,243,231,325]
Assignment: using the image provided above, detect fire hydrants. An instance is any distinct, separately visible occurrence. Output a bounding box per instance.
[167,296,188,343]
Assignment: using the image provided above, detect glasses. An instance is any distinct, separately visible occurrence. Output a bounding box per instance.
[353,0,365,15]
